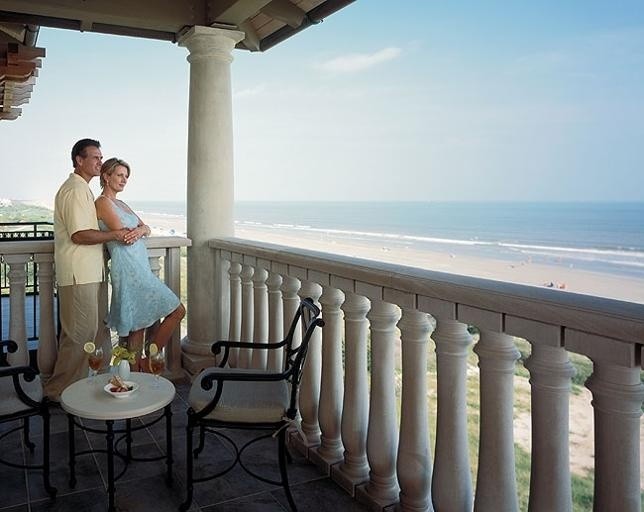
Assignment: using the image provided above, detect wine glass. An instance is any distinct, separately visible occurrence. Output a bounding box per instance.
[88,352,102,385]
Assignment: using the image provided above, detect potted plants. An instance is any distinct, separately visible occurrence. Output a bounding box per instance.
[113,346,138,380]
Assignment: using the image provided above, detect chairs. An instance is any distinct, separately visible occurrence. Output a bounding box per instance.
[1,340,58,499]
[184,297,325,511]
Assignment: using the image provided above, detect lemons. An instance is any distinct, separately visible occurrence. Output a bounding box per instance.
[149,343,158,355]
[84,342,96,354]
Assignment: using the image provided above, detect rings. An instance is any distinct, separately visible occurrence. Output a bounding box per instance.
[135,236,137,240]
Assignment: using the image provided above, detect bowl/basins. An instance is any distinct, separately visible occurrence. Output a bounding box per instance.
[104,381,139,398]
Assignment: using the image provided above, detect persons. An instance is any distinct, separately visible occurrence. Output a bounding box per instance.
[93,157,186,374]
[42,138,135,402]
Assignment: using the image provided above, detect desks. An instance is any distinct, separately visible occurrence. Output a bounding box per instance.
[60,372,176,511]
